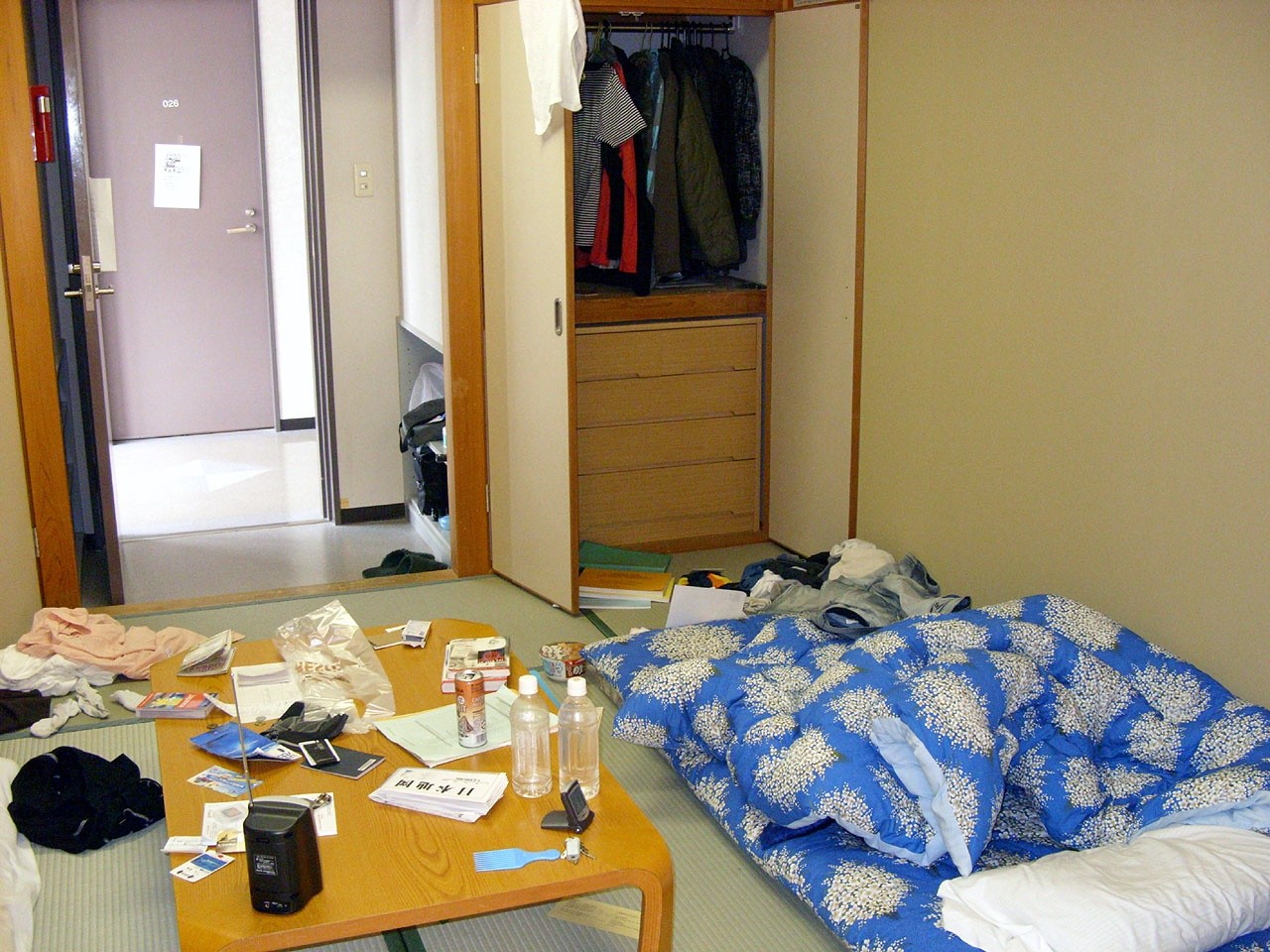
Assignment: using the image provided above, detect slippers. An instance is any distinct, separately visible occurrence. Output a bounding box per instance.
[373,555,448,576]
[362,549,435,580]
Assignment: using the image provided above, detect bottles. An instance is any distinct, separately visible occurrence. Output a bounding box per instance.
[509,675,553,798]
[557,677,600,800]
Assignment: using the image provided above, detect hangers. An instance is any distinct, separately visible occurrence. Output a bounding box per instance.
[585,20,733,63]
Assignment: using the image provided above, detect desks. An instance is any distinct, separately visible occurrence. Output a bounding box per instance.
[151,617,674,952]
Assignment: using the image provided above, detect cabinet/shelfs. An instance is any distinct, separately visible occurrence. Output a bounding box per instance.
[474,0,872,614]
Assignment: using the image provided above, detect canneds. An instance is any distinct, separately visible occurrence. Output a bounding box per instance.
[455,669,488,748]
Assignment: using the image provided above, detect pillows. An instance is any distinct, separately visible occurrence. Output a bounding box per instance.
[937,823,1270,952]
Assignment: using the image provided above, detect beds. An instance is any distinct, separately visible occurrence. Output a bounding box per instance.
[581,593,1270,952]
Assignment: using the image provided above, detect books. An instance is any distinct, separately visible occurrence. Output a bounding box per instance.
[575,538,689,611]
[230,661,305,725]
[440,635,512,694]
[136,691,218,720]
[176,630,237,676]
